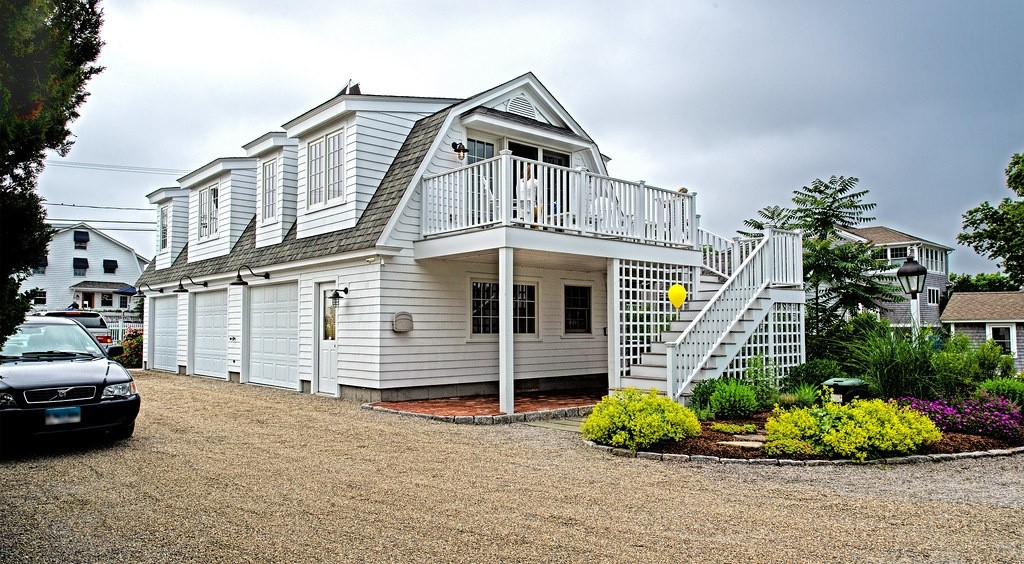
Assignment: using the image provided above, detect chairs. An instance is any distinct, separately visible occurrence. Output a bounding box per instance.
[480,177,530,228]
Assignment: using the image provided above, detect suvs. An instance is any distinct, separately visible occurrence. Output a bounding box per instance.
[25,304,121,360]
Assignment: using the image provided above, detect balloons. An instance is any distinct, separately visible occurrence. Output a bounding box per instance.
[668,284,687,308]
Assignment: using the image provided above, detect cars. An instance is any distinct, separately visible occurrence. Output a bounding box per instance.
[0,315,142,453]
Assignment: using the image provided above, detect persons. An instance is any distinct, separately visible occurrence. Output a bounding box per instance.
[655,187,688,232]
[64,303,79,310]
[516,163,544,225]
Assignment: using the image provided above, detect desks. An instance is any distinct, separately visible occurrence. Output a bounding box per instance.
[546,211,603,238]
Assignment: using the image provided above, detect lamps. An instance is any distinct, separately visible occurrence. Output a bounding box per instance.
[133,284,164,298]
[229,265,270,286]
[452,142,470,160]
[76,295,79,300]
[328,287,349,300]
[172,275,208,292]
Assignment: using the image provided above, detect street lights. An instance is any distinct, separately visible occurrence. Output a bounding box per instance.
[896,256,928,350]
